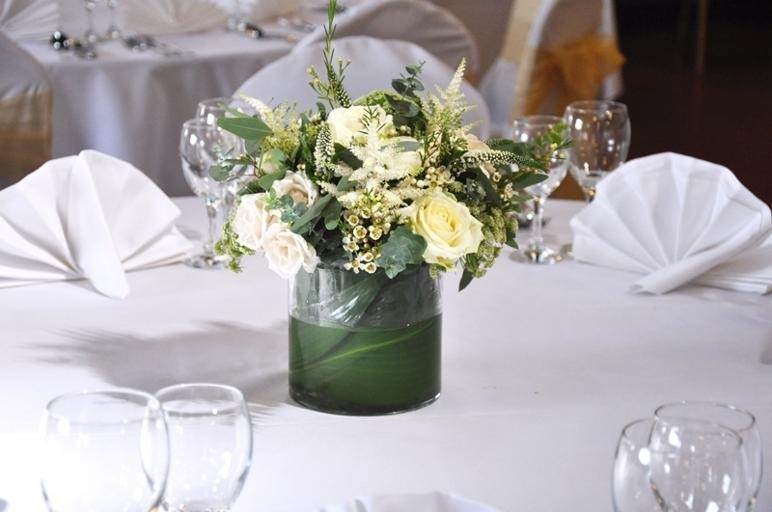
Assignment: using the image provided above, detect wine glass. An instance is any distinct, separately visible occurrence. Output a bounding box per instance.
[180,91,262,271]
[560,99,631,207]
[512,117,569,265]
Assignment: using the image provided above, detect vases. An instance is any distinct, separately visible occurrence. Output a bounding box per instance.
[288,261,443,416]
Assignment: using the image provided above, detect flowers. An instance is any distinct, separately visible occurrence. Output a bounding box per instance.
[210,1,576,291]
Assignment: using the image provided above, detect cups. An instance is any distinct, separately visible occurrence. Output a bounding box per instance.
[610,398,762,511]
[37,381,253,512]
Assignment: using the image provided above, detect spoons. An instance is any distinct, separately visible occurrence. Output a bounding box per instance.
[49,0,345,60]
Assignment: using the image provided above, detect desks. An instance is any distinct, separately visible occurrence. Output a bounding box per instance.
[2,26,319,201]
[0,190,770,510]
